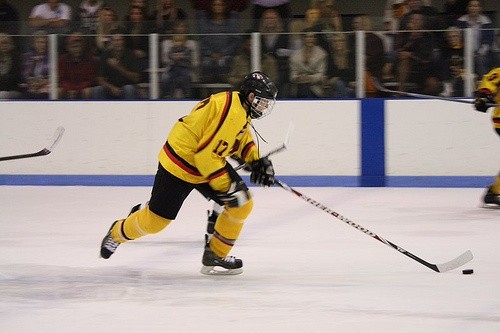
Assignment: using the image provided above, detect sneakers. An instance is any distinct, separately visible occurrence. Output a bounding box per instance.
[484,185,500,209]
[98,220,121,259]
[200,234,243,275]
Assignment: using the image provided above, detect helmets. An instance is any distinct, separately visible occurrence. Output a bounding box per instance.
[239,71,279,120]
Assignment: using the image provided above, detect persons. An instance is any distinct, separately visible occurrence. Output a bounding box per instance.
[100,71,278,275]
[0,0,499,97]
[475,68,500,210]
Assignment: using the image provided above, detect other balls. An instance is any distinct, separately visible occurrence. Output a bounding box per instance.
[462,269,474,274]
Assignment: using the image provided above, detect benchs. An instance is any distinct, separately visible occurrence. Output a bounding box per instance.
[139,77,234,99]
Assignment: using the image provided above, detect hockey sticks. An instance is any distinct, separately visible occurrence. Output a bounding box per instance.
[369,69,500,108]
[274,176,474,274]
[0,126,66,162]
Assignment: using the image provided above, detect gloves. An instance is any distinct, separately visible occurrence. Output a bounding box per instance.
[472,94,491,113]
[219,181,251,207]
[250,158,275,188]
